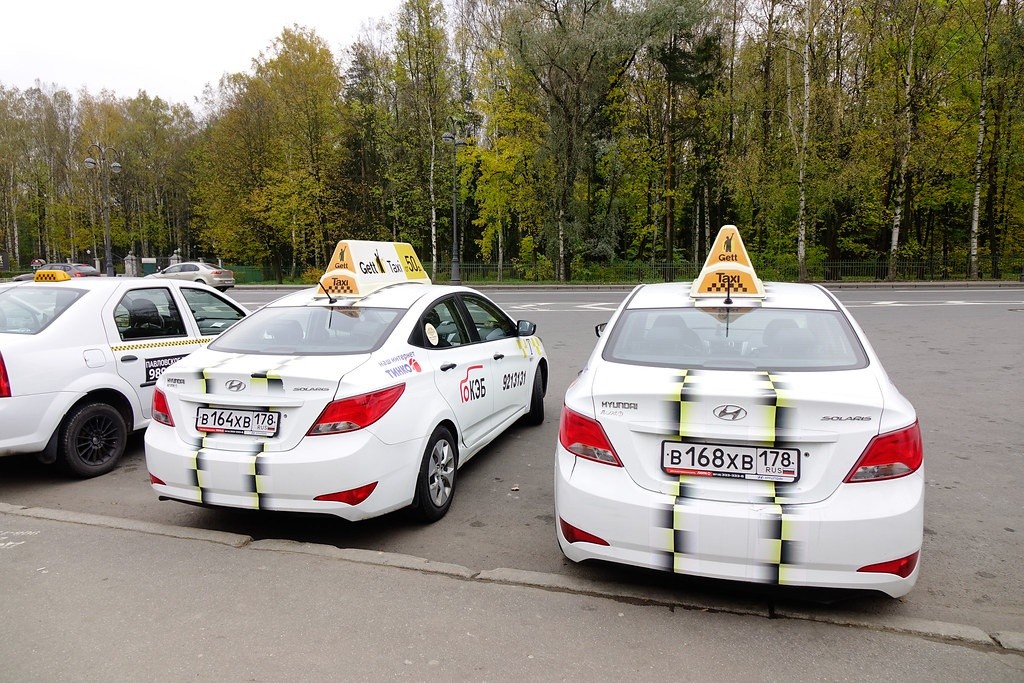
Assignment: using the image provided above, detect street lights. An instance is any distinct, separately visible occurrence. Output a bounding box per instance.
[82,142,123,277]
[440,112,471,296]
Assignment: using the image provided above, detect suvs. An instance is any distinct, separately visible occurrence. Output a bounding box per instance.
[13,263,102,281]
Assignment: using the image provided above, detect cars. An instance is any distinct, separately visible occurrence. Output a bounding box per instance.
[556,280,924,599]
[0,277,252,475]
[145,283,549,522]
[144,262,235,292]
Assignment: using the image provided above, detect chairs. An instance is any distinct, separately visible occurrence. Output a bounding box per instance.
[745,318,829,359]
[121,298,177,338]
[640,315,709,357]
[265,318,313,343]
